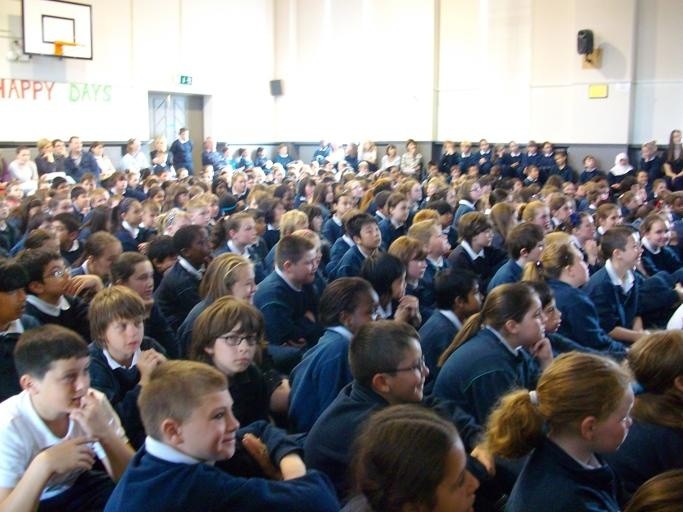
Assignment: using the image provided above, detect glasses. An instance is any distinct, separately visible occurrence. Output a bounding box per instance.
[43,267,72,279]
[217,335,258,346]
[375,355,426,374]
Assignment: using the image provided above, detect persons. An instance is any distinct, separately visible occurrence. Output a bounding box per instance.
[624,469,683,512]
[191,295,292,427]
[339,403,481,512]
[87,285,169,450]
[432,282,554,453]
[103,359,341,512]
[0,324,136,512]
[609,328,683,512]
[287,277,380,433]
[303,320,496,509]
[0,127,682,392]
[0,265,41,403]
[484,351,634,511]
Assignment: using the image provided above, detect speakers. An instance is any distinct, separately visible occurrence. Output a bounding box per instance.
[270,79,280,95]
[578,28,597,57]
